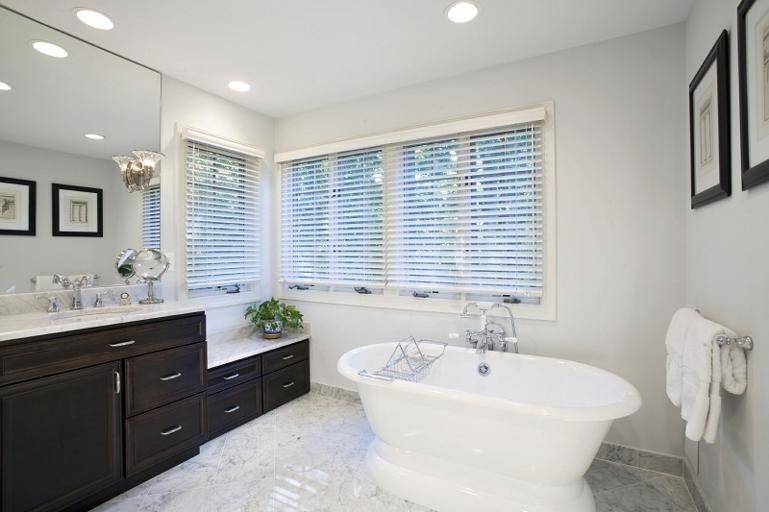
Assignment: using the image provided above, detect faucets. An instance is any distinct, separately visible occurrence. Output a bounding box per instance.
[465,310,488,354]
[65,274,92,311]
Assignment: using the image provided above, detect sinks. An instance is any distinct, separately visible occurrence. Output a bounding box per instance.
[45,306,145,322]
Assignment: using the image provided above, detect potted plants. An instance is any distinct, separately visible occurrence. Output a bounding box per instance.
[245,297,304,339]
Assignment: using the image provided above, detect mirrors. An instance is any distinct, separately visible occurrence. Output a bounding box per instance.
[113,248,137,285]
[0,3,162,296]
[133,247,169,304]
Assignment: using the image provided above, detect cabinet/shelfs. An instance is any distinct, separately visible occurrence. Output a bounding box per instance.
[207,338,263,440]
[263,340,311,415]
[0,306,207,512]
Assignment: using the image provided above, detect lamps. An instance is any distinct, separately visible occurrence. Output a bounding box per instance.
[111,154,134,191]
[126,149,164,191]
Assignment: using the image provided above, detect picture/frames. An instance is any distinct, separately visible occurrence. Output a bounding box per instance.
[52,183,103,236]
[0,177,36,235]
[736,0,769,192]
[688,29,731,208]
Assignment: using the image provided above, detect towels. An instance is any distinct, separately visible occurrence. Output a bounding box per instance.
[64,274,94,290]
[662,304,748,444]
[34,273,64,292]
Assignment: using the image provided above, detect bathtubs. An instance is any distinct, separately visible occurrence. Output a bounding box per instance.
[336,339,643,512]
